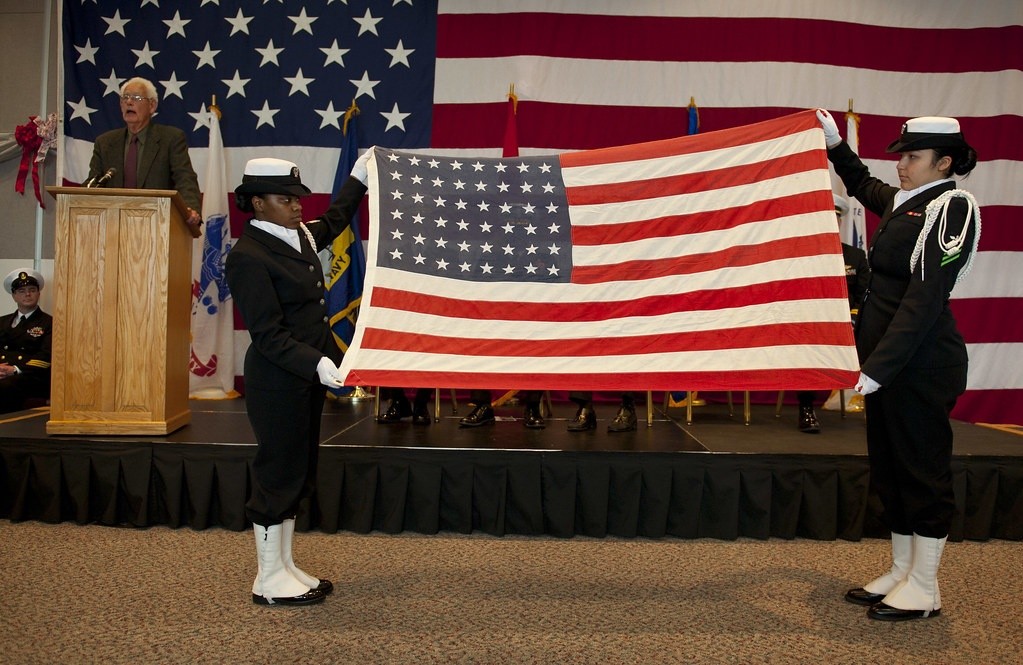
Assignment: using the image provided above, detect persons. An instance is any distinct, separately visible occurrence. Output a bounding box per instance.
[0,268,53,414]
[374,387,431,425]
[800,193,866,432]
[816,108,981,620]
[567,391,638,431]
[228,145,376,607]
[460,389,544,428]
[81,78,202,225]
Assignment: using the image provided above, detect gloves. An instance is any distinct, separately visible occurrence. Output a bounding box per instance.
[351,146,374,180]
[855,373,881,395]
[315,356,344,389]
[813,108,841,146]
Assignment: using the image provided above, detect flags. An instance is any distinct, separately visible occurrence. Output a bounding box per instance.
[57,0,1023,425]
[325,115,365,398]
[670,107,698,407]
[334,109,863,389]
[823,113,867,409]
[189,104,234,394]
[492,97,519,405]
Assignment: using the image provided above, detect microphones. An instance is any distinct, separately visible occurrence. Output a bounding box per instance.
[98,167,117,185]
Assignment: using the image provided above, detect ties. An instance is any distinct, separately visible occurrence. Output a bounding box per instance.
[125,135,137,189]
[16,315,26,327]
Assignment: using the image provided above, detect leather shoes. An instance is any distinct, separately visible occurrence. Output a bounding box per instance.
[373,398,410,423]
[459,404,495,426]
[797,407,820,431]
[412,405,431,424]
[567,407,597,431]
[607,406,638,431]
[524,405,544,428]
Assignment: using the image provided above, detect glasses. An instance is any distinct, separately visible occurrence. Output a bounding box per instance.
[118,94,149,103]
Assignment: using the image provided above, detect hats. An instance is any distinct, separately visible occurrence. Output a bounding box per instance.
[832,194,849,216]
[2,268,45,295]
[233,159,312,196]
[886,117,963,154]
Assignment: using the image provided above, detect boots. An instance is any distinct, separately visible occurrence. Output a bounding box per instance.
[867,531,948,620]
[846,533,914,603]
[252,523,325,604]
[281,515,333,595]
